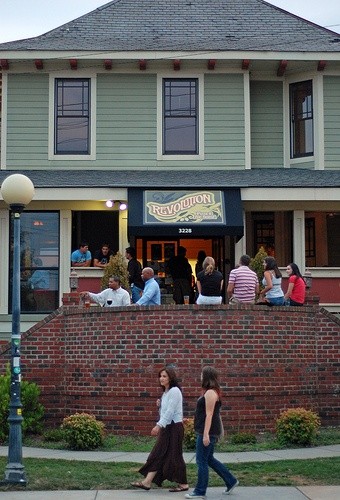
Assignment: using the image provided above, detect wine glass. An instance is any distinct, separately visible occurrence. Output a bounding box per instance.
[99,299,105,307]
[107,298,112,306]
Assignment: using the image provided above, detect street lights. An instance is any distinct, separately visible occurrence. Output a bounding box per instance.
[1,173,35,487]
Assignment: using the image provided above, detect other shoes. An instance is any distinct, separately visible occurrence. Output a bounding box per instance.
[185,493,205,498]
[223,480,239,495]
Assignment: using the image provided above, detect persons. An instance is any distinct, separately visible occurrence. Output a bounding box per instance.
[71,242,91,267]
[255,256,285,306]
[195,250,207,275]
[135,267,160,305]
[80,276,130,307]
[94,244,113,267]
[22,257,49,290]
[184,366,239,499]
[227,255,259,304]
[130,367,189,492]
[196,256,224,304]
[284,263,306,306]
[165,246,196,305]
[125,246,144,303]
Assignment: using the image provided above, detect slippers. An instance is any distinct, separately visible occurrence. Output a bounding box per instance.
[169,485,188,492]
[130,481,150,490]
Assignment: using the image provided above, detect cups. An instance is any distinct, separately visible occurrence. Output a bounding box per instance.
[184,296,190,304]
[85,299,90,307]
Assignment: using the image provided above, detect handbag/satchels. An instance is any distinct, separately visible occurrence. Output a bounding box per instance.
[255,293,273,307]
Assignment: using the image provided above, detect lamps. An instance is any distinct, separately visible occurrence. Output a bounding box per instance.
[105,200,128,210]
